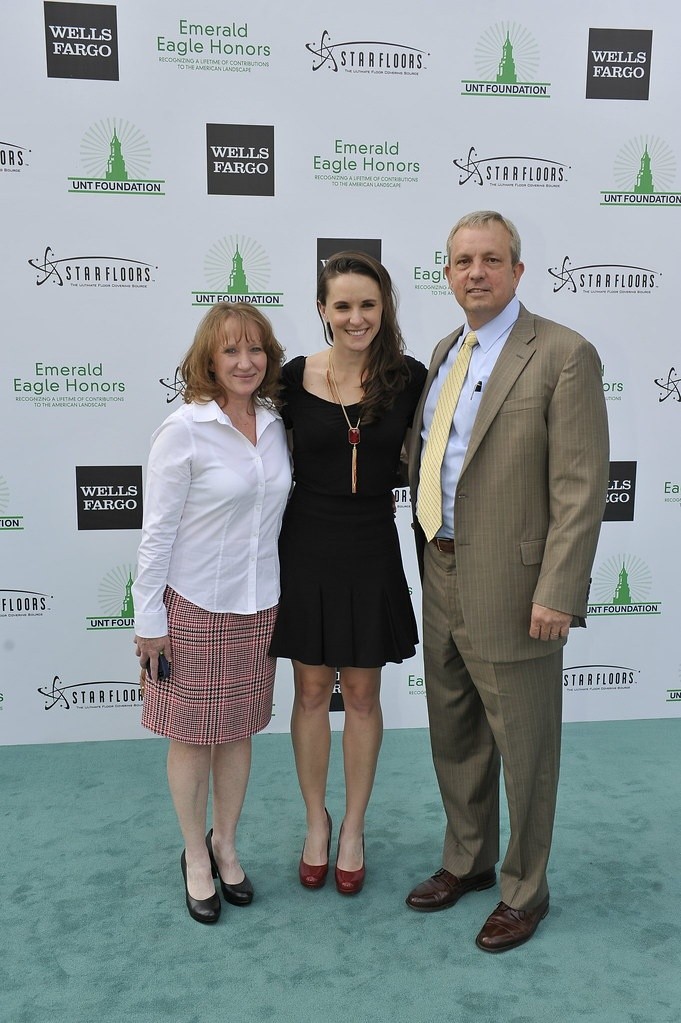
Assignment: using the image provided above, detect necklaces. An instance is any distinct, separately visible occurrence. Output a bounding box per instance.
[327,349,364,494]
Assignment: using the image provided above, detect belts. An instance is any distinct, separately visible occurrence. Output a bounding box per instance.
[429,536,456,553]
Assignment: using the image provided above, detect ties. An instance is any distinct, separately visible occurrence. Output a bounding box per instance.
[415,331,478,544]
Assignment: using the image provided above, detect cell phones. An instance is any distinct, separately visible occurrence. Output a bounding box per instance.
[146,651,169,680]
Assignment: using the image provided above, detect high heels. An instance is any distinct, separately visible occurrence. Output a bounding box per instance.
[300,807,333,890]
[205,828,253,907]
[336,822,366,895]
[180,848,221,923]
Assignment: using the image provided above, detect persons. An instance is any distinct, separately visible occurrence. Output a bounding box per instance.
[253,250,429,895]
[403,210,612,956]
[130,300,292,926]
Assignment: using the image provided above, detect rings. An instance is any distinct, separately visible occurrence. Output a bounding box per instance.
[550,632,559,637]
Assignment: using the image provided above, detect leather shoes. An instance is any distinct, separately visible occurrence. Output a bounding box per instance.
[405,866,497,912]
[475,890,550,953]
